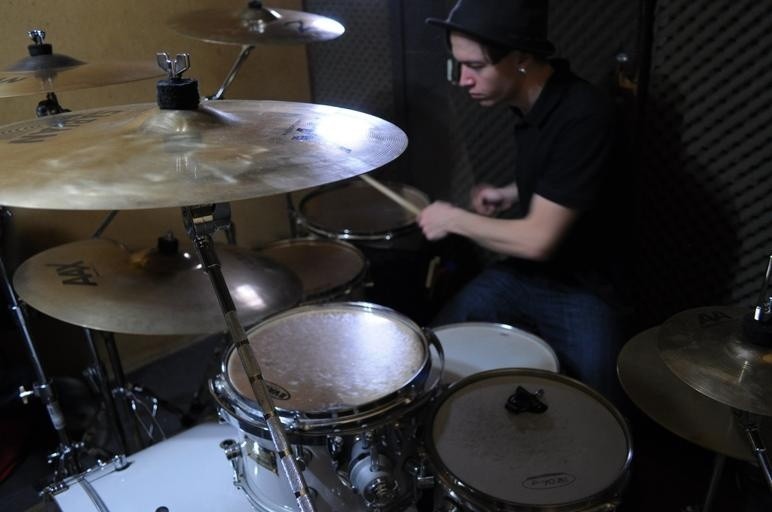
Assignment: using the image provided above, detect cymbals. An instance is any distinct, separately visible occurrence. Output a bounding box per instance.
[656,303,771,416]
[14,236,303,335]
[616,325,771,462]
[164,7,346,46]
[2,97,407,211]
[0,54,168,97]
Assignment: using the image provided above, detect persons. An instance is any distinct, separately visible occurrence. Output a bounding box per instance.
[414,0,627,403]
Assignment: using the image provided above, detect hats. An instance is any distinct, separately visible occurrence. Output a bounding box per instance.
[426,1,556,56]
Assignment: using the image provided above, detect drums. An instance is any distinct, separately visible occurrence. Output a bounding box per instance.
[424,323,559,393]
[252,234,378,304]
[209,298,431,510]
[40,417,365,511]
[295,177,438,313]
[420,368,634,511]
